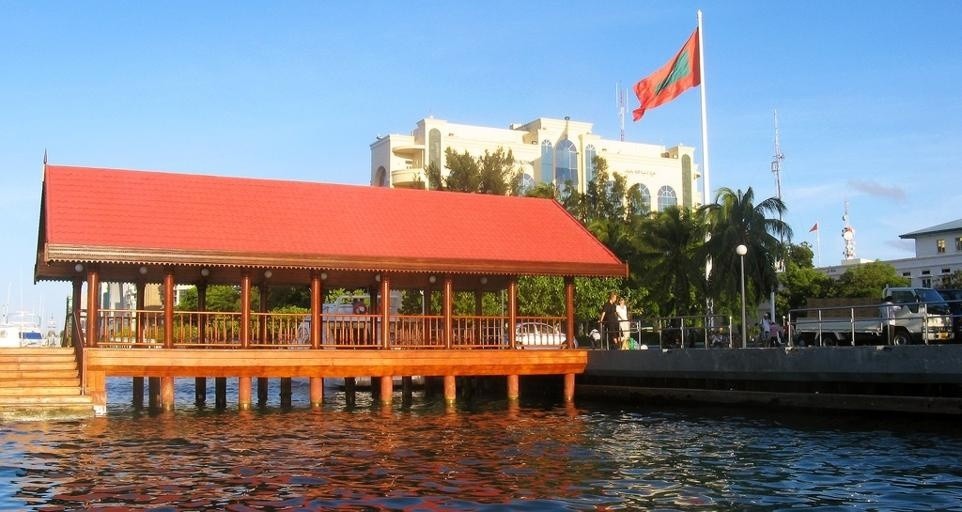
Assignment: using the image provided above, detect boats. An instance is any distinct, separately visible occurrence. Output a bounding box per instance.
[286,303,425,387]
[0,279,47,348]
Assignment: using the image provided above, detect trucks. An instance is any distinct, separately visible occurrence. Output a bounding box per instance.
[796,284,955,347]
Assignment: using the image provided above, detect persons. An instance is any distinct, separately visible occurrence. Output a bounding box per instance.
[586,325,601,349]
[614,295,630,351]
[877,296,902,347]
[630,312,789,348]
[596,291,624,350]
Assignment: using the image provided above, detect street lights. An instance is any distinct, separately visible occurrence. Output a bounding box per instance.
[736,243,748,348]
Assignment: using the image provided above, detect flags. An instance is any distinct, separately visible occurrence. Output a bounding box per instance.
[629,24,699,123]
[809,223,817,232]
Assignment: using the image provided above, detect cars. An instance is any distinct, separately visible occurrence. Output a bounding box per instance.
[502,322,578,350]
